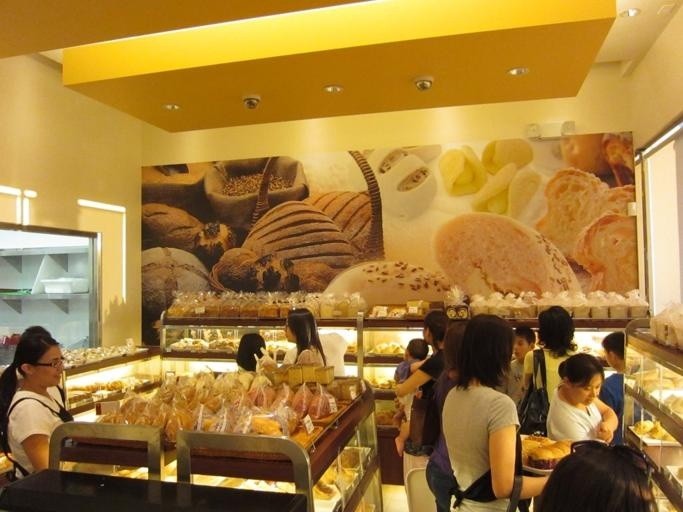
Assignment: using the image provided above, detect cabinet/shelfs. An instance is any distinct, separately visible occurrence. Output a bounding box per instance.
[48,378,222,506]
[176,375,384,511]
[0,227,99,366]
[624,314,683,511]
[469,291,651,371]
[57,344,159,421]
[160,308,364,379]
[343,301,475,485]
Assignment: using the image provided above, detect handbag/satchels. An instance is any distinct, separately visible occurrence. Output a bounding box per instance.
[516,347,551,438]
[404,389,440,457]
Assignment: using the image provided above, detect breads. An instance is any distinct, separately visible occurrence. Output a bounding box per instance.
[560,135,613,176]
[346,343,404,354]
[170,337,238,357]
[634,308,683,441]
[243,202,353,274]
[141,203,202,254]
[266,347,287,361]
[220,249,257,292]
[305,190,371,256]
[324,262,453,303]
[603,135,634,185]
[249,254,298,293]
[368,144,438,219]
[445,299,648,320]
[484,138,534,177]
[113,448,368,500]
[167,298,429,322]
[441,146,487,198]
[374,410,394,424]
[536,168,635,273]
[573,212,640,296]
[141,247,211,345]
[432,212,580,297]
[68,381,97,409]
[98,361,361,450]
[520,435,574,470]
[195,222,238,270]
[290,263,337,294]
[468,164,541,220]
[1,457,12,472]
[368,377,398,389]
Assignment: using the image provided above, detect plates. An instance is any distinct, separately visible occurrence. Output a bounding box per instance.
[518,435,554,475]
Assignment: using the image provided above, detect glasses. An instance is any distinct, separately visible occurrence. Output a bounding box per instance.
[570,439,659,488]
[36,355,67,370]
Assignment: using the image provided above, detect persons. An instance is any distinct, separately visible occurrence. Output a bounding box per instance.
[598,332,624,447]
[441,313,550,512]
[425,321,464,512]
[237,333,266,372]
[284,309,326,368]
[0,331,74,485]
[546,352,619,445]
[533,440,658,512]
[395,338,429,458]
[496,325,537,406]
[24,326,66,410]
[397,311,448,512]
[521,305,578,404]
